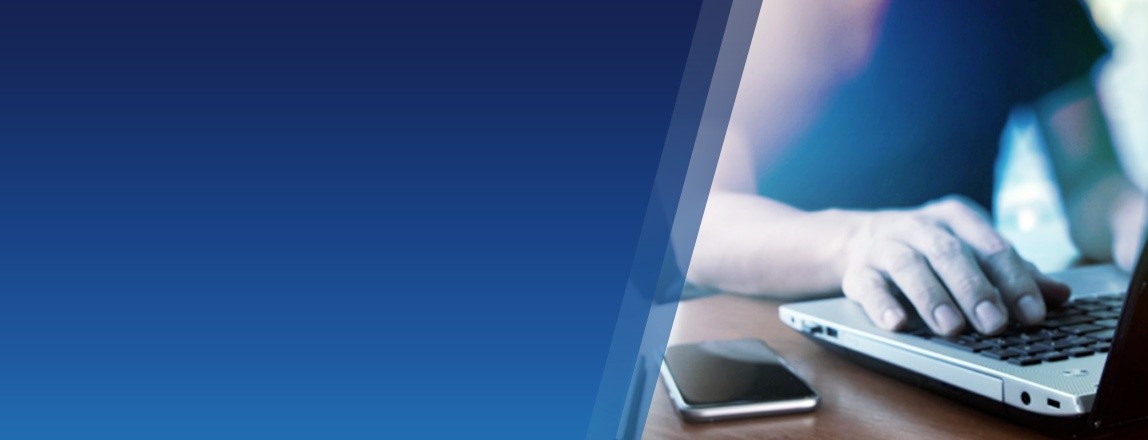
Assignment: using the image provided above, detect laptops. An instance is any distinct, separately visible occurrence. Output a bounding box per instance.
[776,232,1148,437]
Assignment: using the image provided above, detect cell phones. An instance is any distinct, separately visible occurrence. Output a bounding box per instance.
[660,337,821,425]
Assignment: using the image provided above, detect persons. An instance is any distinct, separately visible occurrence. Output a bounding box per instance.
[670,0,1148,339]
